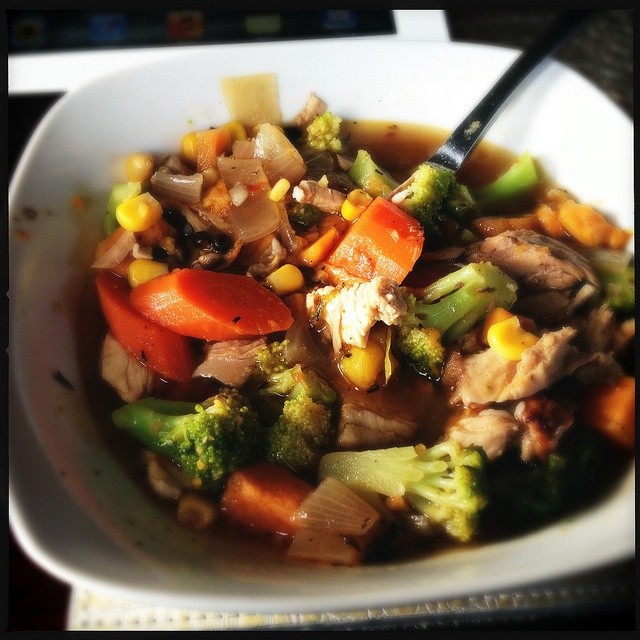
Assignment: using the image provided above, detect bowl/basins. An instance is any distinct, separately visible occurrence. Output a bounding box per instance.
[8,39,634,610]
